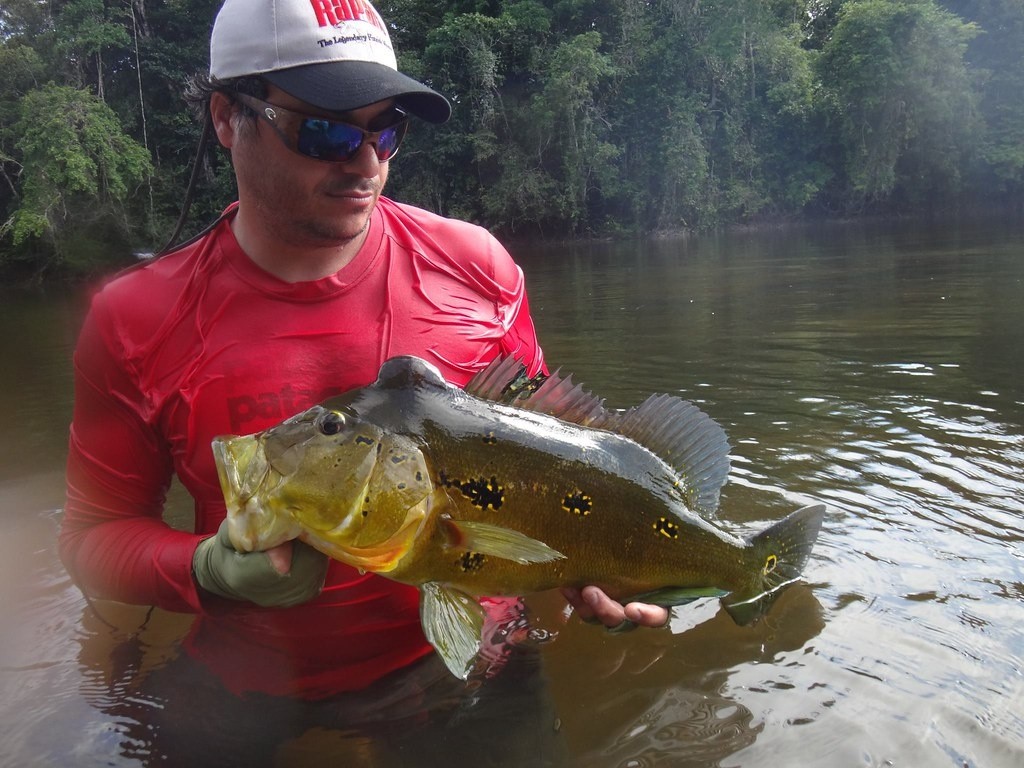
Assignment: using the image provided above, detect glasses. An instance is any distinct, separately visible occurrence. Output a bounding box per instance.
[223,88,411,162]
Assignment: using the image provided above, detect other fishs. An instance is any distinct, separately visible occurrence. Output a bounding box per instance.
[216,351,828,685]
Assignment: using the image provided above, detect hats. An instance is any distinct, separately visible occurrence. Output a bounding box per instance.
[210,0,453,126]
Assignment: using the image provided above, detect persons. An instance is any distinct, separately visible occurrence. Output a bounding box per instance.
[58,0,672,633]
